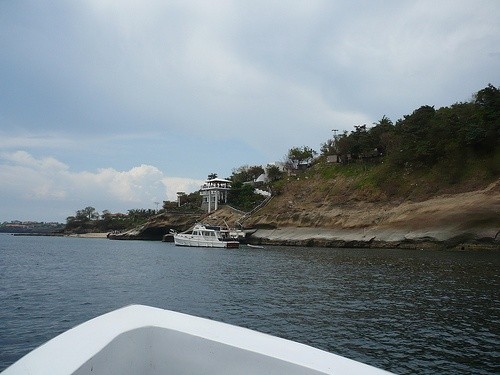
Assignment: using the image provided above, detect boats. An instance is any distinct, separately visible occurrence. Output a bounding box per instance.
[169,217,240,249]
